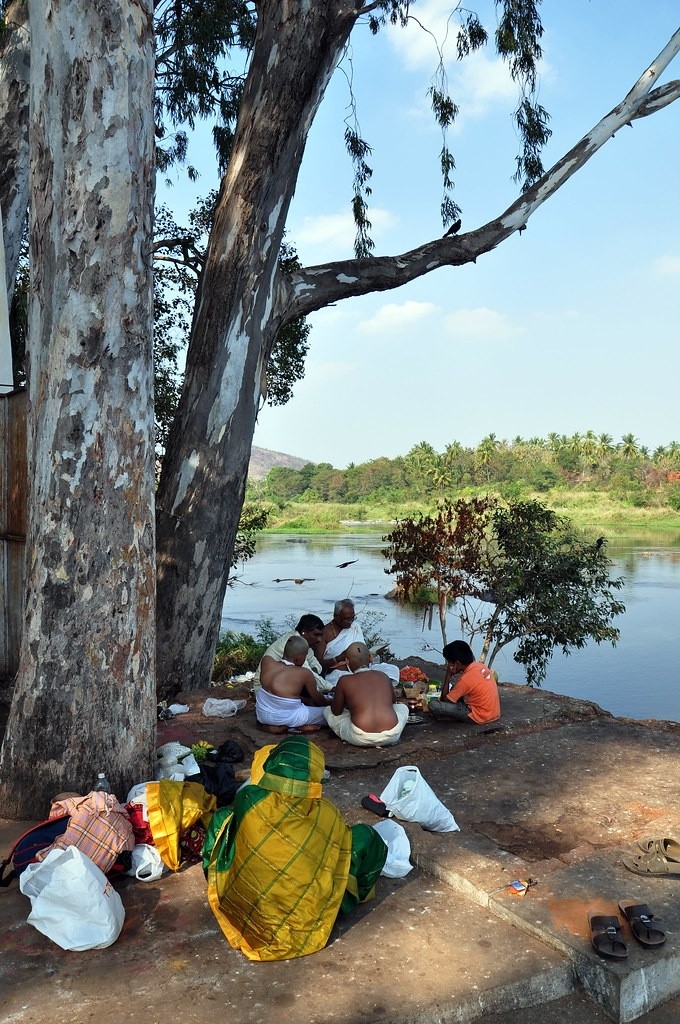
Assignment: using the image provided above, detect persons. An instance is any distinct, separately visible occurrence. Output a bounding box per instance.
[255,635,331,734]
[314,599,400,687]
[427,640,501,725]
[200,737,388,962]
[250,613,336,703]
[323,642,409,748]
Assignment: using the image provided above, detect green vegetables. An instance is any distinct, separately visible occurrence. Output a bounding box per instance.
[178,746,207,764]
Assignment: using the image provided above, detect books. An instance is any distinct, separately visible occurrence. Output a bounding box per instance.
[369,643,391,655]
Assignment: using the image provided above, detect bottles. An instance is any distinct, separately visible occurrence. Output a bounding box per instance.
[94,773,112,796]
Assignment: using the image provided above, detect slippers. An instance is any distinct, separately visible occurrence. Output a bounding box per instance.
[618,899,666,949]
[623,838,680,879]
[588,909,629,961]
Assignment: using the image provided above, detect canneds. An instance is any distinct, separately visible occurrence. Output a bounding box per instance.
[162,700,166,707]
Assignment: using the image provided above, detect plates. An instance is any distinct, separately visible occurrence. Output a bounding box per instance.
[407,715,424,724]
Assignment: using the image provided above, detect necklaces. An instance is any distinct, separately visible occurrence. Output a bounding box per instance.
[331,620,337,638]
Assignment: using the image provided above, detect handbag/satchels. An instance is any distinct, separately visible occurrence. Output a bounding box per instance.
[127,843,170,883]
[20,845,125,951]
[379,766,461,833]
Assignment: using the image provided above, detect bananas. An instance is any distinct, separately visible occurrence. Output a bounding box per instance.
[191,740,214,750]
